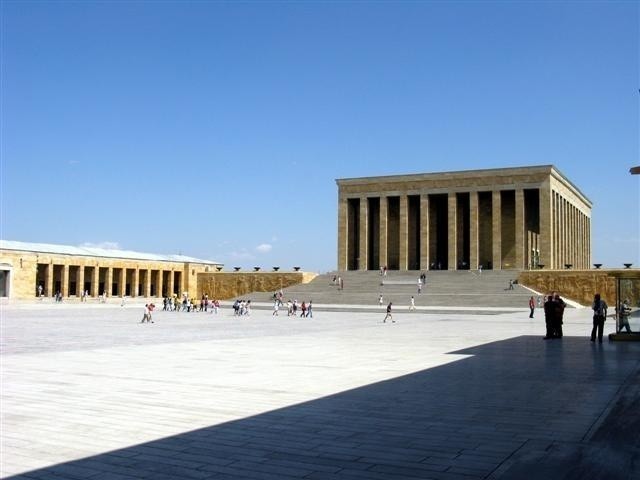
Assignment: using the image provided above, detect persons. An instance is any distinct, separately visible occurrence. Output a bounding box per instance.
[380,267,383,277]
[332,275,337,285]
[378,295,386,306]
[408,296,415,311]
[417,275,425,295]
[506,278,637,344]
[413,261,468,270]
[422,273,426,283]
[383,266,387,277]
[160,290,315,319]
[37,284,108,305]
[478,265,483,273]
[140,303,150,323]
[382,301,396,324]
[337,277,343,291]
[148,302,156,323]
